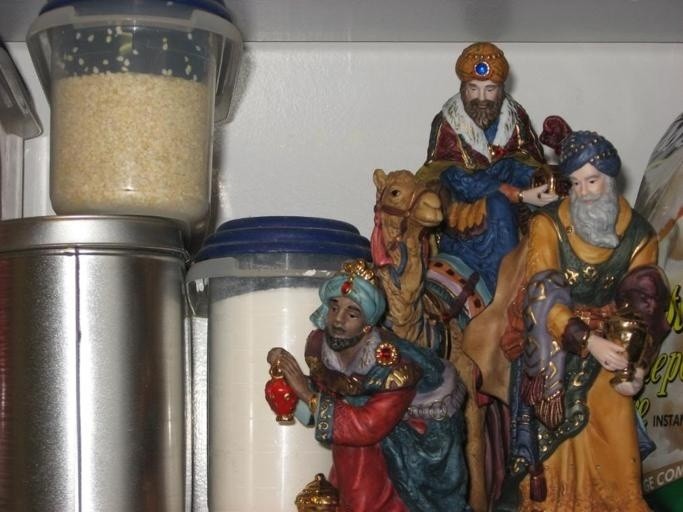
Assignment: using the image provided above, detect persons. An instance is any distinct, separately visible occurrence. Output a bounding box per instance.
[258,257,472,511]
[425,41,572,299]
[495,132,670,511]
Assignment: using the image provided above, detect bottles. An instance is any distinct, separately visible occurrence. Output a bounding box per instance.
[1,215,192,512]
[184,216,373,512]
[25,2,241,222]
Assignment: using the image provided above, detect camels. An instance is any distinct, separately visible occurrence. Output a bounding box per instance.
[370,168,491,512]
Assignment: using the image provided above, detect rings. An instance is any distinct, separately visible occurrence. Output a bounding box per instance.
[604,360,610,365]
[537,192,541,198]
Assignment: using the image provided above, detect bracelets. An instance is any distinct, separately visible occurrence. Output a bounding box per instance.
[517,190,523,203]
[308,393,317,411]
